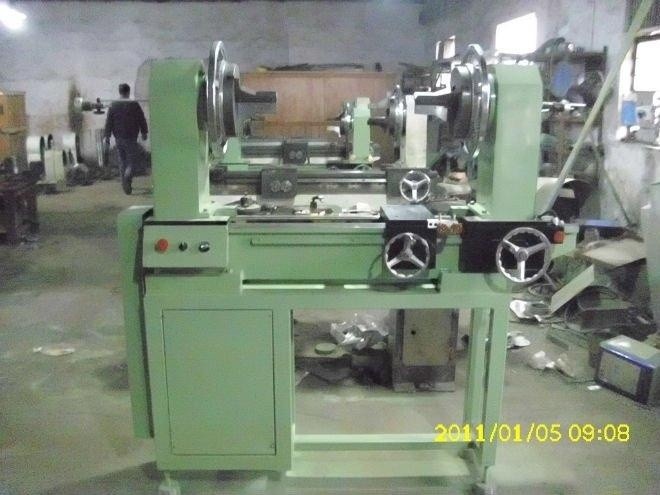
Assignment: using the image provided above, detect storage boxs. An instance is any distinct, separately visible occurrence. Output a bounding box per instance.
[595,334,660,406]
[1,133,31,164]
[0,92,30,130]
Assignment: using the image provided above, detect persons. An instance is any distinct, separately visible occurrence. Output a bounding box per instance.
[101,83,148,194]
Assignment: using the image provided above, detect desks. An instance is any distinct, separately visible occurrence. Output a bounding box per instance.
[1,162,46,246]
